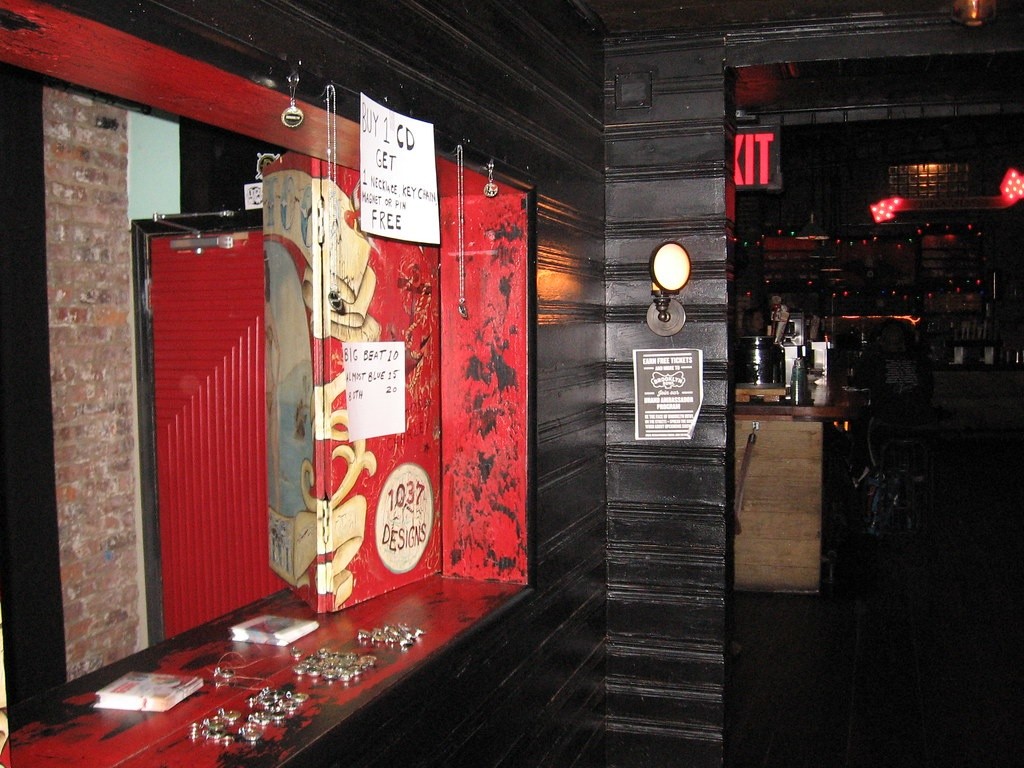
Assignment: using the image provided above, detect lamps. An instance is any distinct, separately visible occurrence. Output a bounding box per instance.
[645,241,688,335]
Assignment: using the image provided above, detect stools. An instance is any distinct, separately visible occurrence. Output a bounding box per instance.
[864,412,941,536]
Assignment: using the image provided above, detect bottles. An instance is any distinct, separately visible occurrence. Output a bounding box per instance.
[790,358,806,406]
